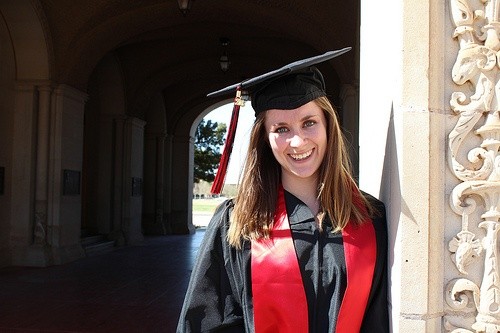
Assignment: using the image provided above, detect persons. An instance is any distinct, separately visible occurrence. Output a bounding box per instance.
[175,47,390,332]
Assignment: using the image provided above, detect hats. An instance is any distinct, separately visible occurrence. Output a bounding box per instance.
[206,46,353,194]
